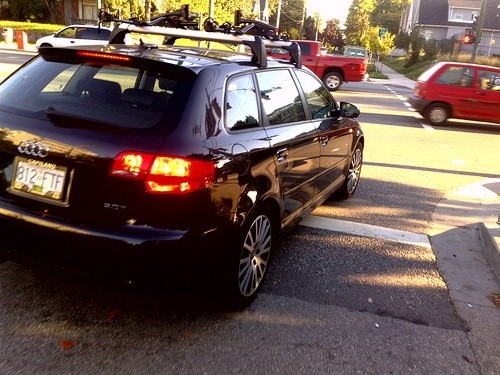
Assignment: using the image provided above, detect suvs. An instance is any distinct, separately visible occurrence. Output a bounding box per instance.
[0,4,365,312]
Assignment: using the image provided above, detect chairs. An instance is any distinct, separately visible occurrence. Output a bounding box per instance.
[159,75,175,93]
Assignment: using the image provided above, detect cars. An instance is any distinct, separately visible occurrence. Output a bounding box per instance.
[408,62,500,126]
[35,25,134,49]
[348,48,364,57]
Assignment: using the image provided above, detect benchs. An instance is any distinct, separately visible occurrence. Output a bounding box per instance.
[79,77,173,120]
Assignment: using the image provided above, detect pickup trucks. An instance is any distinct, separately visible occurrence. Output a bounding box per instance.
[265,39,367,92]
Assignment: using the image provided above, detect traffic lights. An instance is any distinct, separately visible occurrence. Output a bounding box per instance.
[464,36,475,43]
